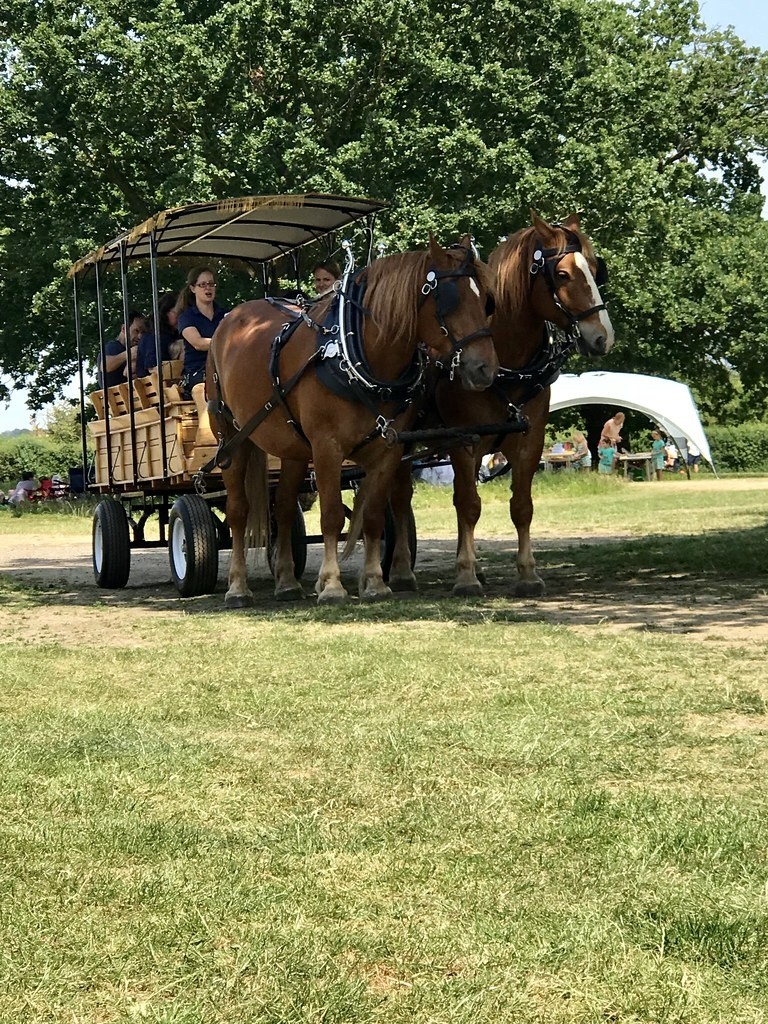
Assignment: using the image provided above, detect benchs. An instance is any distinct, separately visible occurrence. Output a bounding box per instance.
[89,359,353,490]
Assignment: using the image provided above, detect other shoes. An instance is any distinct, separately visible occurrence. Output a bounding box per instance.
[196,428,218,446]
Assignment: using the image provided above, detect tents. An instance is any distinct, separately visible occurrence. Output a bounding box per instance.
[478,371,721,479]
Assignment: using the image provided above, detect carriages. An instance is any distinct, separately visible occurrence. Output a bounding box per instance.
[66,195,616,609]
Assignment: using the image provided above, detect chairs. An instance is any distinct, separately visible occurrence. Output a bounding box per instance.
[23,475,57,503]
[53,468,94,499]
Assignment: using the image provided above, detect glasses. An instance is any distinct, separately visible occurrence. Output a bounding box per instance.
[194,282,217,288]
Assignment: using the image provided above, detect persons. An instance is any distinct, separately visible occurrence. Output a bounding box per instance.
[176,265,231,446]
[98,291,186,389]
[545,412,701,485]
[8,471,53,504]
[313,258,341,293]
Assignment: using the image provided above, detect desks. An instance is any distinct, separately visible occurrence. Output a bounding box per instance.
[545,452,573,471]
[620,455,651,481]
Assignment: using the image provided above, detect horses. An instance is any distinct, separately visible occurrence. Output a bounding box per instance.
[204,207,615,610]
